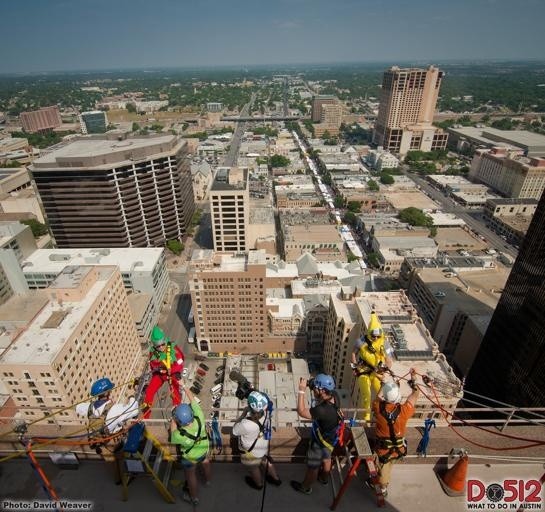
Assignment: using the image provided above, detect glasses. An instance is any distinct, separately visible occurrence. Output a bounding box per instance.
[154,342,165,349]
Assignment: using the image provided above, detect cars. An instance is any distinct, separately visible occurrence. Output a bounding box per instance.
[136,376,150,409]
[193,156,222,170]
[190,352,225,422]
[268,364,274,371]
[183,368,189,377]
[416,178,520,258]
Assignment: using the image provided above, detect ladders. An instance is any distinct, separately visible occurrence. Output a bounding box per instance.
[118,422,182,504]
[329,426,385,511]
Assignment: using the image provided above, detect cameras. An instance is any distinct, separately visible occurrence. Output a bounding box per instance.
[299,377,314,390]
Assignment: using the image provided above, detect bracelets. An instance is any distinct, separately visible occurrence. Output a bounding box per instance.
[297,390,306,395]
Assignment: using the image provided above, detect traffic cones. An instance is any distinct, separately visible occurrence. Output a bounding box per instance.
[436,454,471,498]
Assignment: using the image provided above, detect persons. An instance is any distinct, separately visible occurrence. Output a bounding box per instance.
[365,366,421,496]
[73,377,140,486]
[288,373,341,495]
[230,383,282,490]
[349,312,395,428]
[168,377,213,505]
[140,324,185,419]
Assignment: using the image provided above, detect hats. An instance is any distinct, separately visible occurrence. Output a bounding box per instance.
[151,325,165,342]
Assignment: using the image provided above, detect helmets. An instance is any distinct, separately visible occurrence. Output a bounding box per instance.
[311,373,336,391]
[90,378,115,395]
[248,391,268,412]
[382,380,400,403]
[175,404,195,426]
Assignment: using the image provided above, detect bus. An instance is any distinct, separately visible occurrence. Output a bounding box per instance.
[188,327,196,345]
[188,305,195,324]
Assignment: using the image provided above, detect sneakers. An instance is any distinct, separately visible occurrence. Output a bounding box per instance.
[183,492,199,505]
[200,478,210,486]
[367,478,388,496]
[266,476,282,487]
[244,475,263,490]
[317,471,329,486]
[289,480,312,495]
